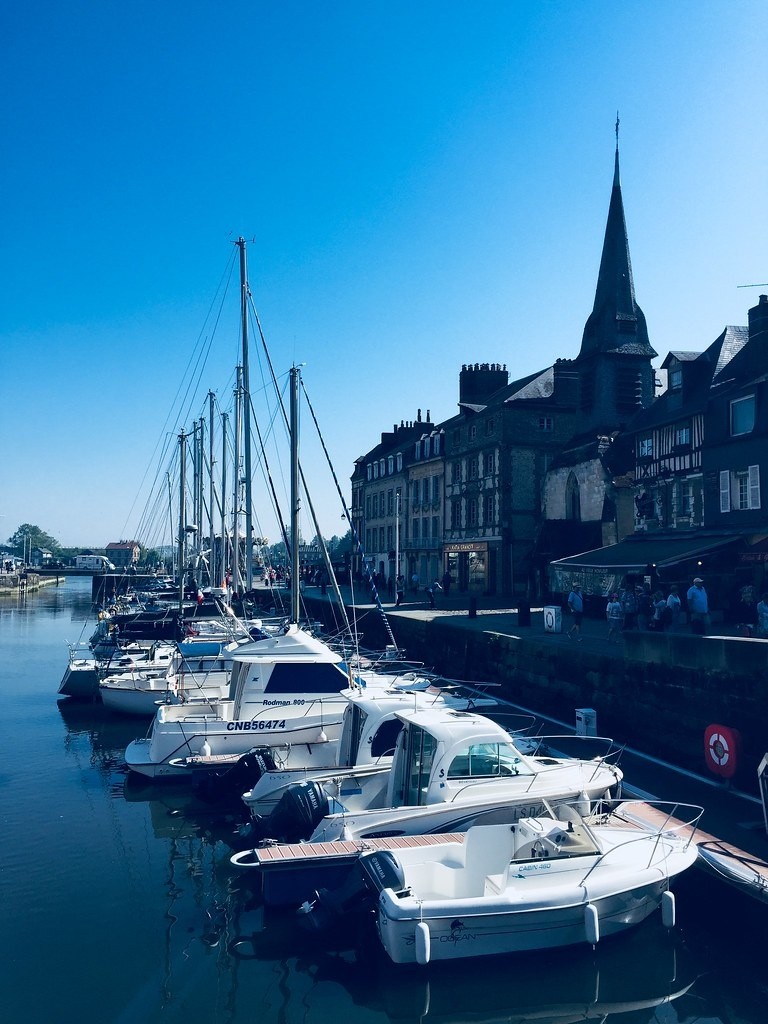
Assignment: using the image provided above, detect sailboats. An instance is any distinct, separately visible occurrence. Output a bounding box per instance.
[57,232,504,713]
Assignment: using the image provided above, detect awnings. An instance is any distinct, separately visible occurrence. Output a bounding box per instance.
[550,535,742,597]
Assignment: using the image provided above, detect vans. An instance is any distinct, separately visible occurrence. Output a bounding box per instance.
[76,555,115,572]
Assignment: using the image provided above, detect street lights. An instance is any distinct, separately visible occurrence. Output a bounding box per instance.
[395,492,400,604]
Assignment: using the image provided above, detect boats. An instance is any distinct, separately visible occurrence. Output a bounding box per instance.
[0,533,66,596]
[123,569,627,844]
[252,797,706,972]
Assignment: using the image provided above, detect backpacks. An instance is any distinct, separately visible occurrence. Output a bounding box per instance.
[644,596,656,616]
[662,606,673,626]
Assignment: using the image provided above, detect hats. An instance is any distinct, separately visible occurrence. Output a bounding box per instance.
[611,594,618,598]
[693,578,704,583]
[573,581,581,588]
[635,586,642,590]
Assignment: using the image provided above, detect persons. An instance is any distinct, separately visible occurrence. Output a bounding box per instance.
[687,577,712,635]
[757,593,768,635]
[622,583,650,631]
[395,575,404,608]
[667,585,682,632]
[567,582,583,641]
[426,577,442,609]
[650,591,667,632]
[411,571,419,596]
[606,593,622,641]
[363,571,394,602]
[260,564,328,595]
[441,570,452,597]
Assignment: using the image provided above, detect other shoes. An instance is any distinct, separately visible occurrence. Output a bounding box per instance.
[607,637,609,641]
[324,593,326,595]
[321,592,324,595]
[613,638,617,641]
[429,607,437,610]
[395,605,399,607]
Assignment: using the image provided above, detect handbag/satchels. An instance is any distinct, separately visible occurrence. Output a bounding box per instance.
[569,609,574,616]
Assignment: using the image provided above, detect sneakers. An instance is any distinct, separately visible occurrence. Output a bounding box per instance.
[575,636,583,642]
[566,631,572,640]
